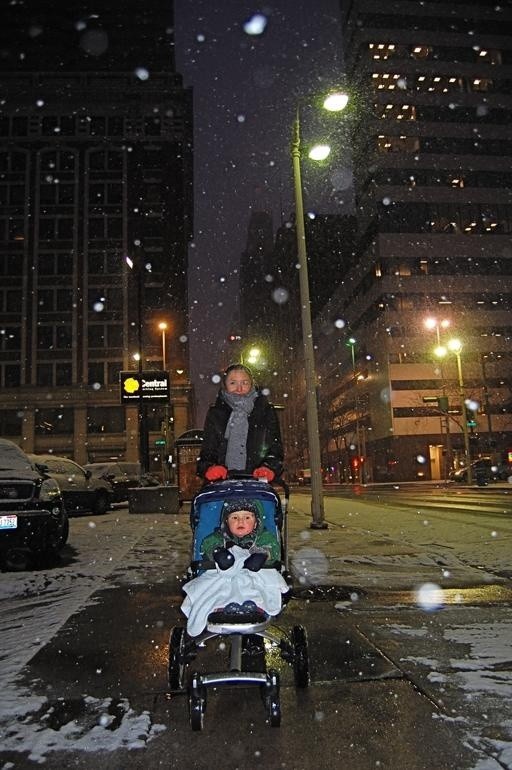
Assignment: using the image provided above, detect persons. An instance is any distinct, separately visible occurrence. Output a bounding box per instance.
[196,362,286,489]
[199,497,283,618]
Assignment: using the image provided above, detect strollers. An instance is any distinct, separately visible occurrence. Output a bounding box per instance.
[167,474,310,731]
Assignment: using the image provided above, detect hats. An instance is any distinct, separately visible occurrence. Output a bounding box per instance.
[224,499,260,519]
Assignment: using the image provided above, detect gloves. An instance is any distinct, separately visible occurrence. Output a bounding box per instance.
[252,466,275,481]
[242,552,265,572]
[213,548,236,571]
[203,466,229,482]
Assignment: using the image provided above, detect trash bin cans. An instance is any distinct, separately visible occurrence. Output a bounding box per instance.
[474,464,486,486]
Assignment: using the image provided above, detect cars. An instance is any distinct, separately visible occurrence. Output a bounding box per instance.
[0,438,141,557]
[449,456,512,482]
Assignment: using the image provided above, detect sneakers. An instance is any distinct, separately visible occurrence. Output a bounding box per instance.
[247,638,265,657]
[228,646,247,660]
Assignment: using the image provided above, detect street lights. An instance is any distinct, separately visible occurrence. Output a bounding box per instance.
[290,86,350,527]
[126,252,149,474]
[349,337,364,484]
[241,343,261,367]
[424,316,471,485]
[158,322,171,445]
